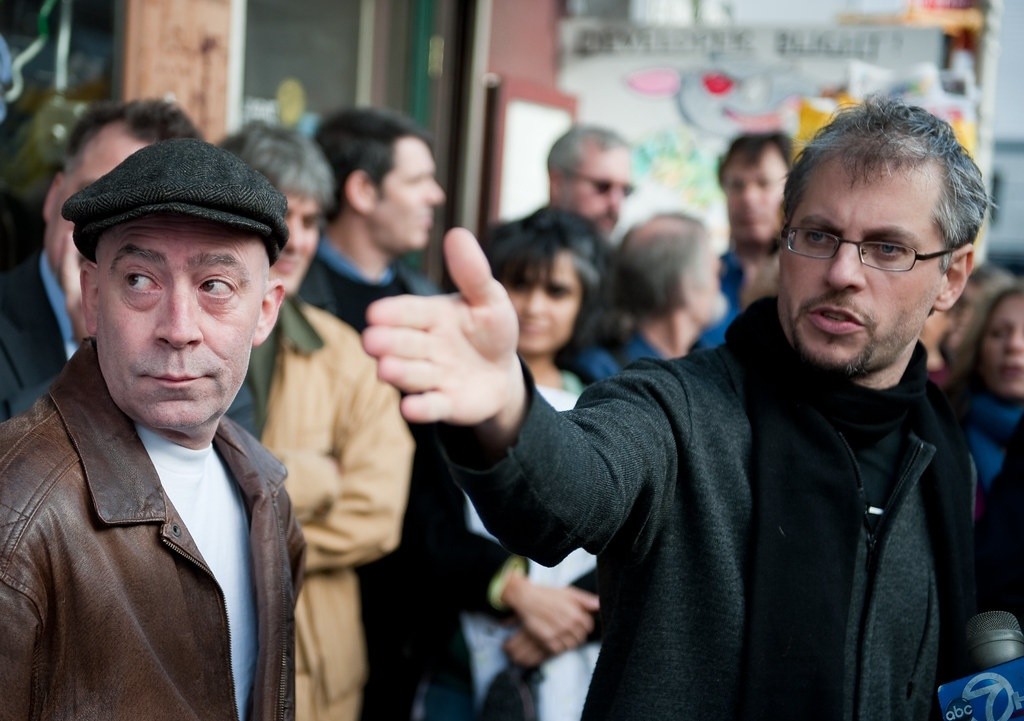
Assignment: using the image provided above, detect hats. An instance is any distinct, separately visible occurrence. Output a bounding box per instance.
[61,138,290,268]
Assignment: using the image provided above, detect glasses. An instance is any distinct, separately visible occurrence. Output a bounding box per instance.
[785,221,958,272]
[572,173,634,201]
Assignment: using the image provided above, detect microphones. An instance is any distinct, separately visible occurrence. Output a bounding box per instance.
[937,610,1024,721]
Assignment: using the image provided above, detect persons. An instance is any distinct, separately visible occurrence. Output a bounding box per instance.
[360,100,978,721]
[0,140,308,721]
[215,119,416,721]
[463,123,1024,721]
[0,101,199,421]
[297,109,466,721]
